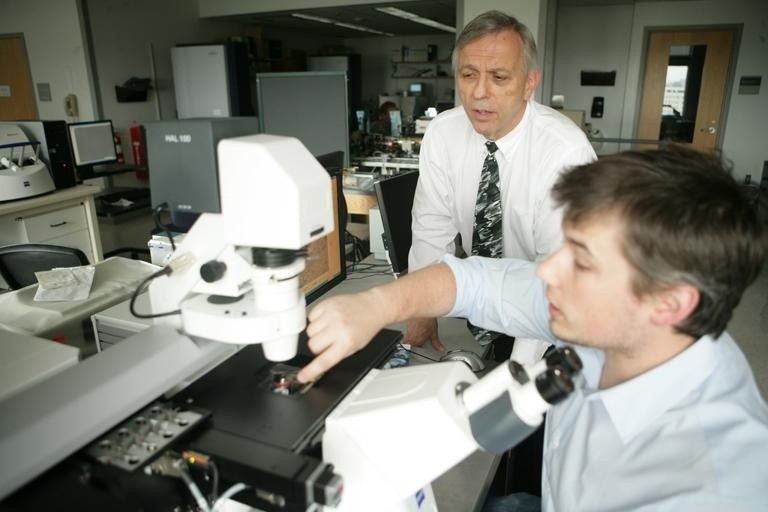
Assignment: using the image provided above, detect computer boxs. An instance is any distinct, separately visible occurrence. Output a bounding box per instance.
[0,120,76,189]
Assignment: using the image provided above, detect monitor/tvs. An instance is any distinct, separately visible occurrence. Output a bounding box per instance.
[298,166,346,305]
[373,171,419,276]
[67,119,118,174]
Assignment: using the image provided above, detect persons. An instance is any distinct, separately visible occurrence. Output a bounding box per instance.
[403,8,602,493]
[372,101,398,135]
[349,101,361,143]
[295,142,768,512]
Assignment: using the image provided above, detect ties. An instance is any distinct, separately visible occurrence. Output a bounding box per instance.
[471,140,506,259]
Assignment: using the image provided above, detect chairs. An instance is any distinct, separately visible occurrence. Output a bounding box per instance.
[0,244,91,289]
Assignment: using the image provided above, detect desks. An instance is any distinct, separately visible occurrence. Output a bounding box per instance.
[74,160,150,223]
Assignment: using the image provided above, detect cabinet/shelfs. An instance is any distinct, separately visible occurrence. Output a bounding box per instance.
[0,182,107,263]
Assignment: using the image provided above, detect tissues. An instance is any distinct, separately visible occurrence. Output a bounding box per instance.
[414,108,438,135]
